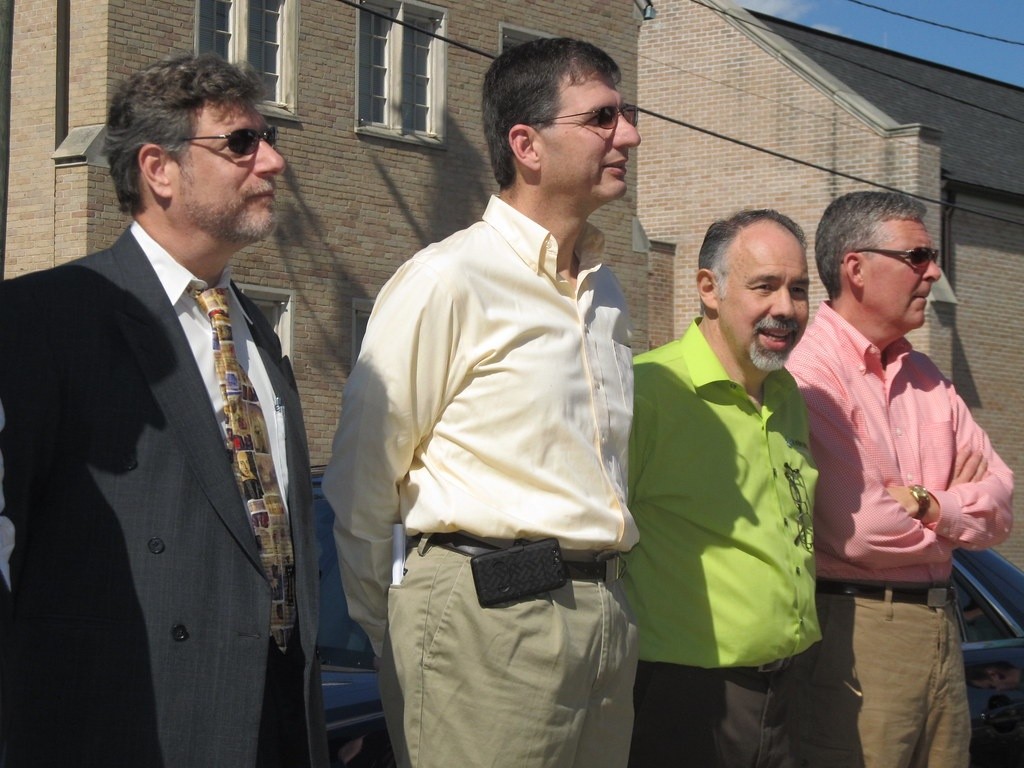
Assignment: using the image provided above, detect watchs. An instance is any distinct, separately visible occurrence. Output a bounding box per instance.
[909,484,931,520]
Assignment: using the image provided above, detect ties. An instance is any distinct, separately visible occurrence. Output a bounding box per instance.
[184,279,296,654]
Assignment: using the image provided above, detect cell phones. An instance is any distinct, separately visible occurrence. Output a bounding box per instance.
[470,538,568,610]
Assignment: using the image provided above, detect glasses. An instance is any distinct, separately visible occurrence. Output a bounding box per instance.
[157,126,276,155]
[841,247,939,268]
[526,104,639,130]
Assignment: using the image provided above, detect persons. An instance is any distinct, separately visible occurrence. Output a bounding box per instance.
[320,35,649,768]
[634,207,820,768]
[1,50,336,768]
[786,188,1016,768]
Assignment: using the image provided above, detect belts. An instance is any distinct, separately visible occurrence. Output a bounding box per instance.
[412,527,621,584]
[812,582,959,606]
[748,653,797,674]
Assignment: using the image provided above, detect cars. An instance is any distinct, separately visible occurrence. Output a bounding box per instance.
[298,467,1024,768]
